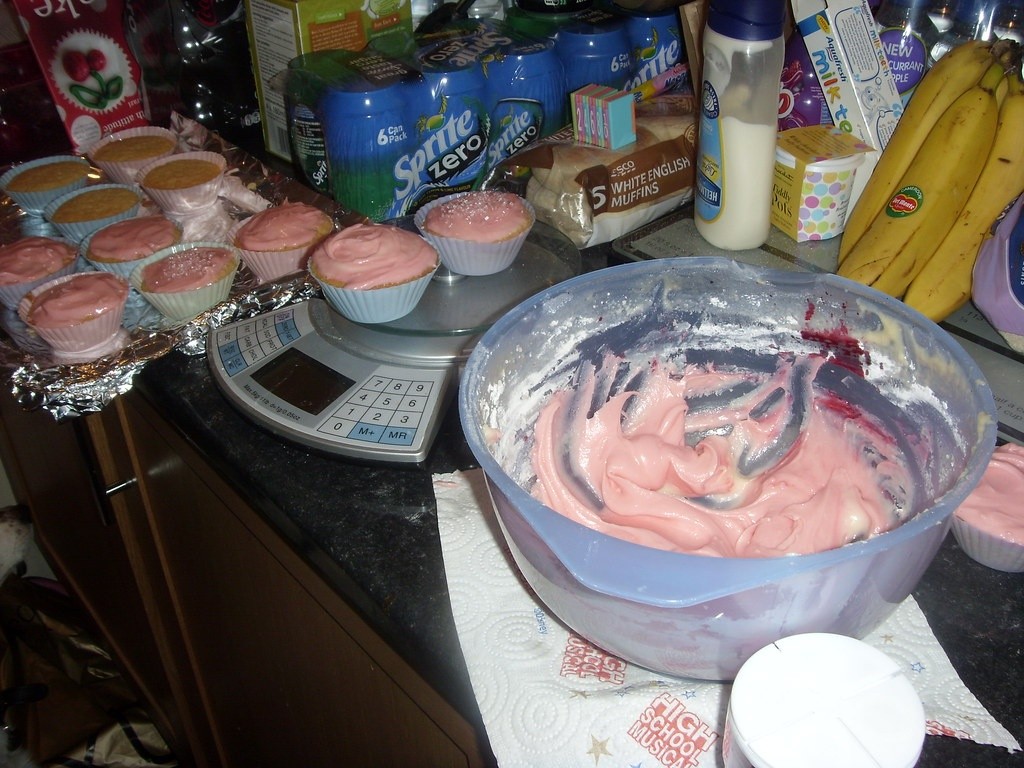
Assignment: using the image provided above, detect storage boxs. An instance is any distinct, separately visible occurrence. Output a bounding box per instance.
[14,0,183,156]
[791,0,905,159]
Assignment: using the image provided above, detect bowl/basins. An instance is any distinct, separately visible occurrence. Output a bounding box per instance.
[1,126,535,363]
[722,633,926,766]
[457,258,998,684]
[951,508,1024,573]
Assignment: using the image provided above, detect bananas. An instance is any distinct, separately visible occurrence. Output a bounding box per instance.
[836,34,1024,328]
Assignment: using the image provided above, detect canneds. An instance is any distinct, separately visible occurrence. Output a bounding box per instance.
[288,0,688,227]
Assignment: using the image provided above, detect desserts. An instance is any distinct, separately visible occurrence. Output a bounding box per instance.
[0,126,335,355]
[306,190,536,324]
[949,442,1024,573]
[518,352,911,680]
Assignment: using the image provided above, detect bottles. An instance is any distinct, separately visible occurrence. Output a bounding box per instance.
[694,1,1024,251]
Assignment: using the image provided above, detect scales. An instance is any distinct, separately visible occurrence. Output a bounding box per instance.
[207,219,593,467]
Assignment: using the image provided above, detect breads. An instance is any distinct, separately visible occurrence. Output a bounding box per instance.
[521,114,700,249]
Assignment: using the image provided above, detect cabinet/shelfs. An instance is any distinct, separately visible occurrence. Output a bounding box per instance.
[244,0,413,161]
[0,330,486,768]
